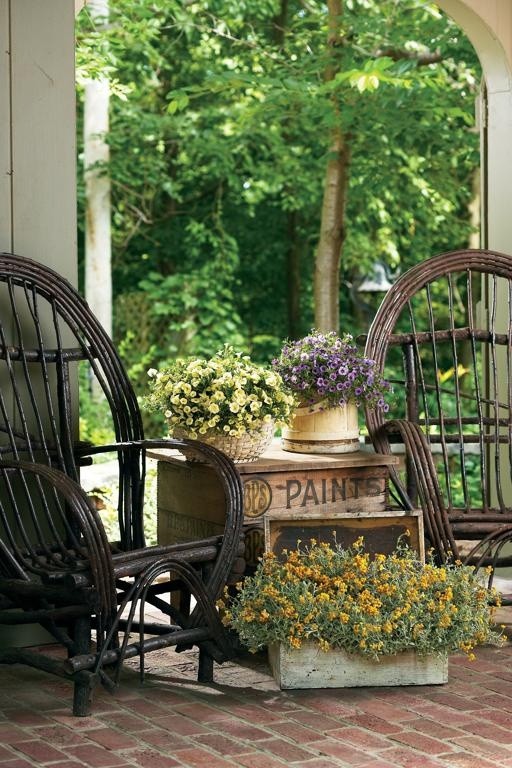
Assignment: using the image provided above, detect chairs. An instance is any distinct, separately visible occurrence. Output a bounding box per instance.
[0,245,243,719]
[358,243,512,601]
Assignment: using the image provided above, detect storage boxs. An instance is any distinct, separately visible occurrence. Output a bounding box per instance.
[260,505,452,689]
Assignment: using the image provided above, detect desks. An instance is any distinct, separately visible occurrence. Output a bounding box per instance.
[144,444,403,639]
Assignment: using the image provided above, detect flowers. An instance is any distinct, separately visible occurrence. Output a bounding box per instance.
[216,531,509,663]
[267,323,397,414]
[141,341,302,438]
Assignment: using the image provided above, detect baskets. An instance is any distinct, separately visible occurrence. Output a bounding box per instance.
[173,422,277,464]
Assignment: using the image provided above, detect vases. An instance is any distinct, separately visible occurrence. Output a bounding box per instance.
[278,396,365,456]
